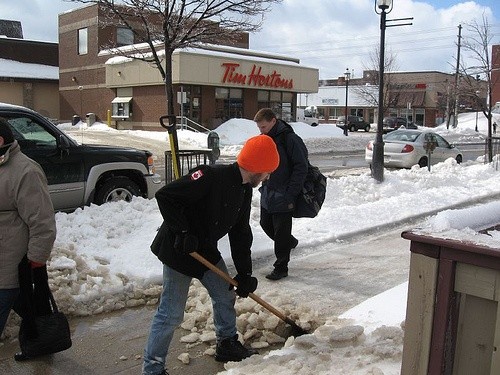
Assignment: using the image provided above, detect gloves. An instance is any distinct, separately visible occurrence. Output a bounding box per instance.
[228,272,259,298]
[173,229,199,256]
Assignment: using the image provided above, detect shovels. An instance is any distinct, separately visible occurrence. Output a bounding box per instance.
[190,251,308,336]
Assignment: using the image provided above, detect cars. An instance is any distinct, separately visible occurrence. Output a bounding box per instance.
[365,128,463,169]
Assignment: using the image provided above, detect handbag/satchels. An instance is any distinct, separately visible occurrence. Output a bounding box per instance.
[18,277,73,359]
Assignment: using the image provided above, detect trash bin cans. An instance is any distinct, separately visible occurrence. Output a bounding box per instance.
[72,115,80,126]
[86,112,96,127]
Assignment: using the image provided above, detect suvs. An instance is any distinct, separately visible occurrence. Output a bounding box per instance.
[0,102,154,214]
[336,115,370,132]
[382,116,418,135]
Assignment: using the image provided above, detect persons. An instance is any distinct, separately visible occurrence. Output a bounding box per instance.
[0,116,56,361]
[253,108,308,280]
[141,135,280,375]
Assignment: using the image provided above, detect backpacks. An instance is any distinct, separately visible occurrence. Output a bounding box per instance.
[293,162,327,218]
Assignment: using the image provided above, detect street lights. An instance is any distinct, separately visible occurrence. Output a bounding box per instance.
[371,0,393,183]
[343,68,351,136]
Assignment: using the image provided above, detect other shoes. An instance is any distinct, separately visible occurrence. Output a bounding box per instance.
[265,267,288,280]
[15,351,24,361]
[215,341,259,364]
[291,235,298,249]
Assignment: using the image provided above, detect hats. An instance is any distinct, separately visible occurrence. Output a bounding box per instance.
[237,133,280,173]
